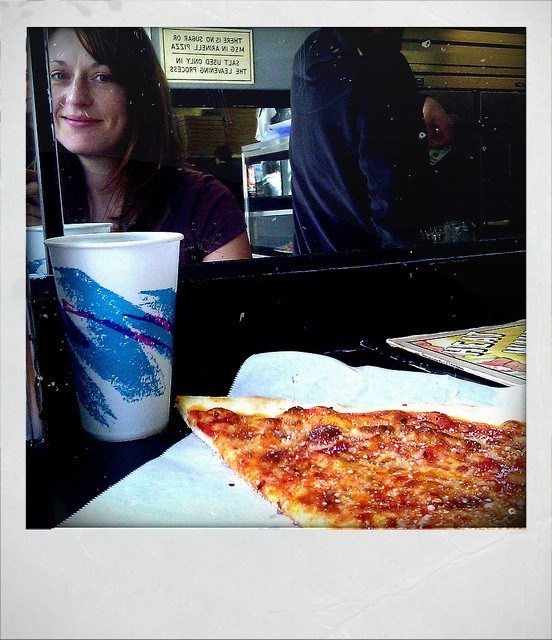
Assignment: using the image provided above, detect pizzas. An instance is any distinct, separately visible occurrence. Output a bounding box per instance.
[178,397,526,528]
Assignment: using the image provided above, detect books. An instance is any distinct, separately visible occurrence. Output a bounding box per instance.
[385,315,526,389]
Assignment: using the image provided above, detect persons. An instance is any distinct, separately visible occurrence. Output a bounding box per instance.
[210,145,245,212]
[49,28,250,266]
[247,166,258,198]
[254,161,282,197]
[26,47,44,227]
[289,26,456,252]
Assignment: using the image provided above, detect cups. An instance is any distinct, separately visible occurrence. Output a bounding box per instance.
[27,222,115,276]
[44,231,186,443]
